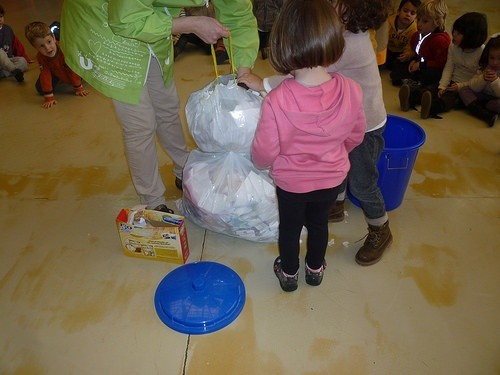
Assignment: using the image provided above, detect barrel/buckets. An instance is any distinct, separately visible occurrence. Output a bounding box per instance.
[347,114,426,212]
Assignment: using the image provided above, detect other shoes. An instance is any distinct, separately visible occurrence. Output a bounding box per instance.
[215,38,229,65]
[145,204,174,214]
[399,84,415,111]
[175,177,182,189]
[13,68,24,81]
[420,90,436,119]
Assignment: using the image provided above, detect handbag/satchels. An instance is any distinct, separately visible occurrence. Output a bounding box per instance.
[184,31,265,155]
[173,146,309,243]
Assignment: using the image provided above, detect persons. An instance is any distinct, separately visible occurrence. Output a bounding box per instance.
[60,0,259,217]
[368,16,390,72]
[252,0,283,58]
[24,22,89,108]
[0,4,34,82]
[458,33,500,127]
[236,0,395,266]
[251,0,367,292]
[174,0,230,66]
[391,0,451,87]
[383,0,422,69]
[400,12,488,119]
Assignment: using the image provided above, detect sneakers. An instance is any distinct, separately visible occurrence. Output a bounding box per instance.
[328,204,344,222]
[274,255,298,292]
[354,220,393,266]
[305,255,327,286]
